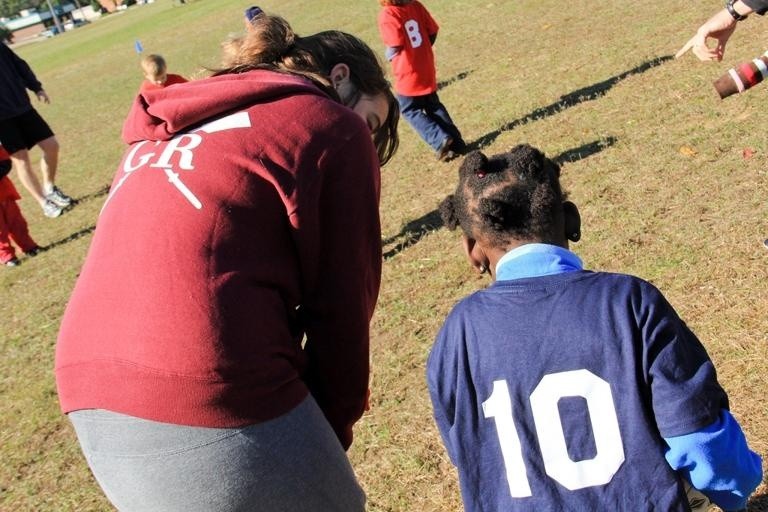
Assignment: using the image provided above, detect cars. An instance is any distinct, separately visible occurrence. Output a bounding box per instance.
[41,18,90,38]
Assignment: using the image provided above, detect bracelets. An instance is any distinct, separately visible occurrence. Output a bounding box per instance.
[726,0,748,20]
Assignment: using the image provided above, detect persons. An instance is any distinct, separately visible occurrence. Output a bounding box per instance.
[141,54,187,89]
[0,143,47,266]
[56,14,400,512]
[675,0,768,62]
[425,145,762,512]
[378,0,466,161]
[0,41,73,219]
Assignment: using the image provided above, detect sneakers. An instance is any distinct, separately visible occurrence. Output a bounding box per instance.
[6,255,22,269]
[38,184,73,218]
[437,131,466,165]
[22,242,45,257]
